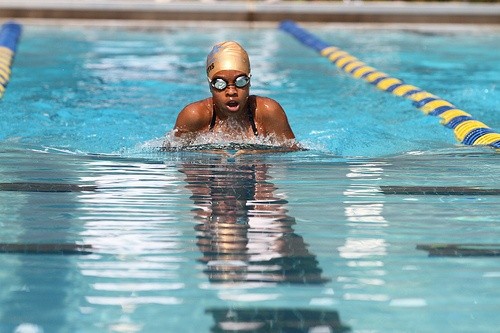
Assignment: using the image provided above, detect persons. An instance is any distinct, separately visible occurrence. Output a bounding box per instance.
[173,41,302,158]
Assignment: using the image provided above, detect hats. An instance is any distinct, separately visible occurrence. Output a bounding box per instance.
[206,40,251,85]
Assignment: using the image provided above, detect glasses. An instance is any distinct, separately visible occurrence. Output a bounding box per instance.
[206,73,251,90]
